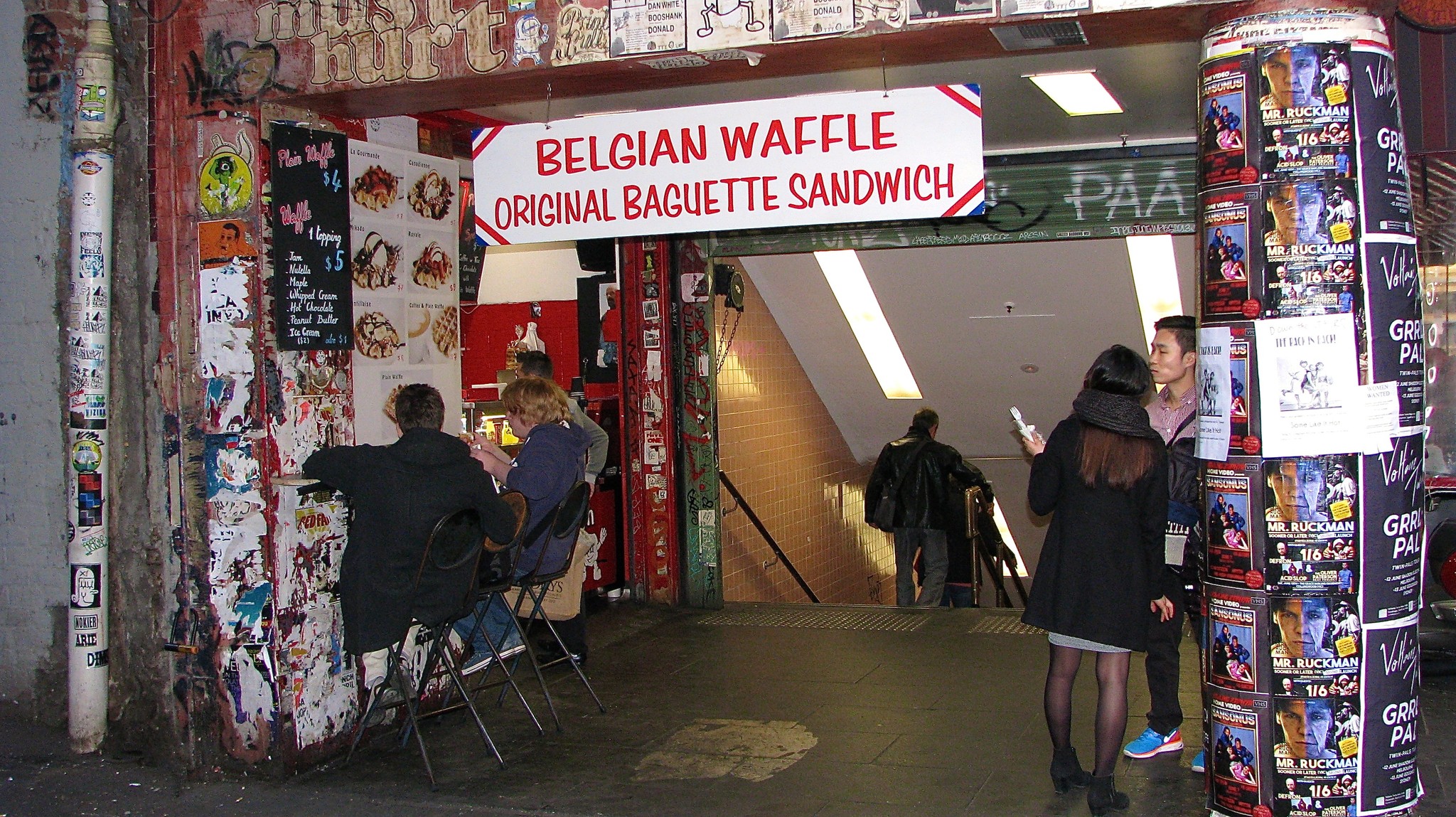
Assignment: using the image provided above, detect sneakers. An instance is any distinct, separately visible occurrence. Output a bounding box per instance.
[1123,726,1184,758]
[1190,750,1204,772]
[512,639,525,655]
[461,647,515,675]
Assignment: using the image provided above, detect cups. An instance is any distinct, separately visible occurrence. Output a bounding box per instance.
[569,377,585,400]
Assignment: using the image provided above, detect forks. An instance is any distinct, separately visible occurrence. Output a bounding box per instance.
[1009,406,1026,429]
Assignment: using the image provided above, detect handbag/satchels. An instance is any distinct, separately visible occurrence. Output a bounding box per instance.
[872,481,896,532]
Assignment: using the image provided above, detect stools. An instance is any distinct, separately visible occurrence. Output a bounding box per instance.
[345,483,606,790]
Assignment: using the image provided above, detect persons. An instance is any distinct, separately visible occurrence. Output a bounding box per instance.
[1286,774,1357,817]
[1021,344,1175,817]
[1206,227,1246,281]
[1335,147,1350,179]
[1266,130,1298,176]
[301,384,517,729]
[1258,45,1351,111]
[1209,495,1249,551]
[452,375,593,676]
[1274,696,1359,759]
[1212,624,1253,683]
[1123,315,1209,773]
[1263,180,1355,317]
[914,509,1018,608]
[1205,98,1244,152]
[1215,726,1257,785]
[1266,455,1359,758]
[864,406,995,608]
[515,350,609,666]
[1230,371,1246,416]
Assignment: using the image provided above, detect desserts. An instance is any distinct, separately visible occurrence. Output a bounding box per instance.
[350,165,459,419]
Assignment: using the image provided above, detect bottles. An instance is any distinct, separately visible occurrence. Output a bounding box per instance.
[485,419,519,445]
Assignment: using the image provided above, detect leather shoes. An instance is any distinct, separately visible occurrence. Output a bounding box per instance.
[536,649,585,667]
[536,638,561,650]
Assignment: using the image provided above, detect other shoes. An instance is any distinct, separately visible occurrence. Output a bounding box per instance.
[360,676,398,727]
[389,654,417,700]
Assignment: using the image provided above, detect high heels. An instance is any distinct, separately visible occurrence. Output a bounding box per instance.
[1087,768,1130,817]
[1050,747,1092,794]
